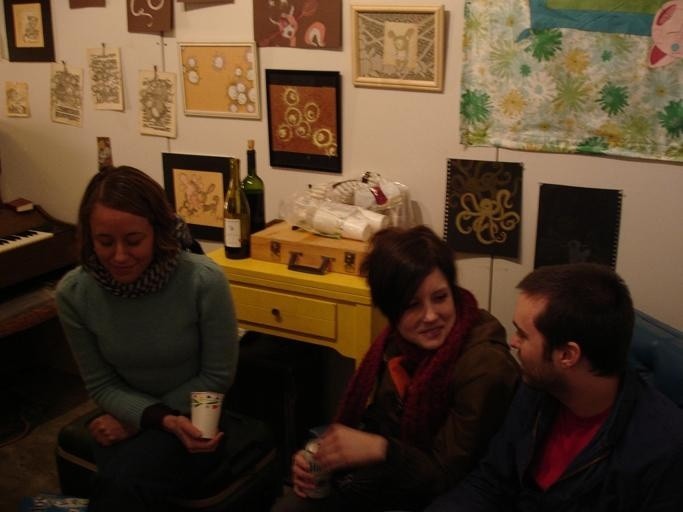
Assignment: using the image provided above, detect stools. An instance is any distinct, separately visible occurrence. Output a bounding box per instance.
[56,408,283,511]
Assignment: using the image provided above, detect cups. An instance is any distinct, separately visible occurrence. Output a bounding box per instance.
[311,204,389,240]
[187,390,225,439]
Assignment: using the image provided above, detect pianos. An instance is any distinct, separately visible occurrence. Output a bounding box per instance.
[0,197,80,340]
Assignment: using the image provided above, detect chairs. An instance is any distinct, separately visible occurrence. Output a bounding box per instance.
[625,307,683,409]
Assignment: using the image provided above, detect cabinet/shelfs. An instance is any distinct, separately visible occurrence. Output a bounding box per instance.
[203,246,389,370]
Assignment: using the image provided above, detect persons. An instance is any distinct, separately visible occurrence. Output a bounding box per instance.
[53,164,238,458]
[266,224,525,509]
[421,260,682,511]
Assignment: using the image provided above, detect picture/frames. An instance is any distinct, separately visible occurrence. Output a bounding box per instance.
[162,153,240,242]
[350,4,446,93]
[176,42,262,120]
[3,0,56,63]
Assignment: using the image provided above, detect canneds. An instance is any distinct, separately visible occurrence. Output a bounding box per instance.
[303,438,333,499]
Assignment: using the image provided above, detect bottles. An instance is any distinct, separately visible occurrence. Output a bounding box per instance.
[242,149,264,229]
[222,160,251,259]
